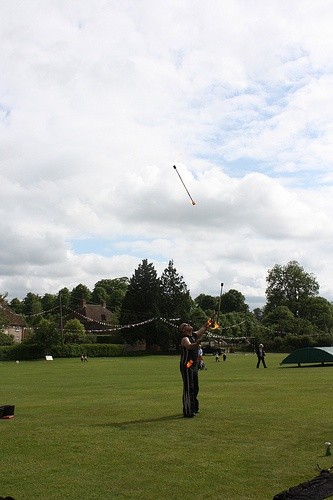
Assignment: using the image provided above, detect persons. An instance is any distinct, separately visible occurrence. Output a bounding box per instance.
[215,352,219,363]
[256,344,268,368]
[85,354,88,362]
[197,344,207,371]
[180,318,215,418]
[81,354,84,362]
[222,351,227,361]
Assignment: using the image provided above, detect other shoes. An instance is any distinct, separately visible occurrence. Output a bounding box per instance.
[185,414,196,417]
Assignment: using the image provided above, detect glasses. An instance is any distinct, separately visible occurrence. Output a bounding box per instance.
[181,323,188,330]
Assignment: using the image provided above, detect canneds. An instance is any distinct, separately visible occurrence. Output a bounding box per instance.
[325,441,331,456]
